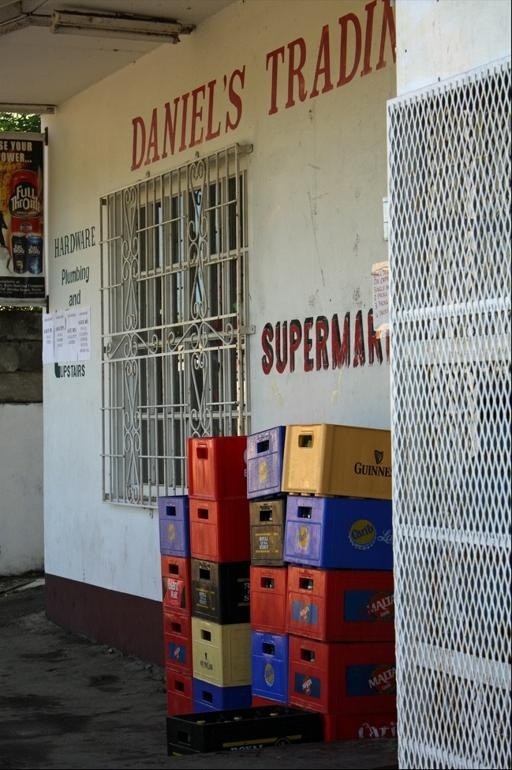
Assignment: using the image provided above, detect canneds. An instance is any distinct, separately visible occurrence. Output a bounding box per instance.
[12,229,42,274]
[10,170,43,257]
